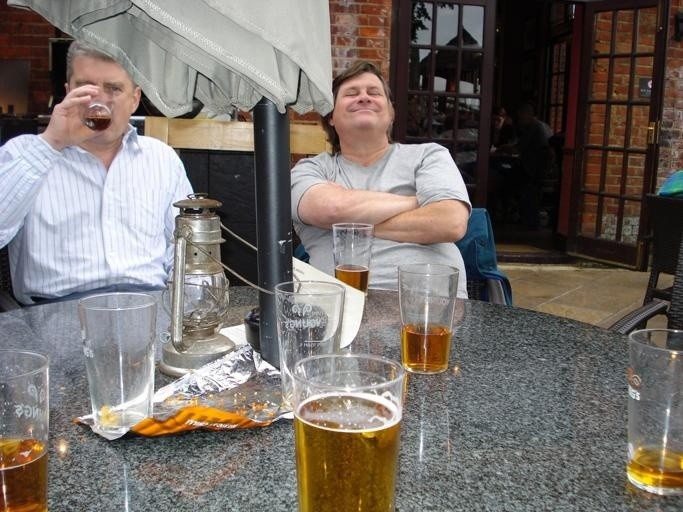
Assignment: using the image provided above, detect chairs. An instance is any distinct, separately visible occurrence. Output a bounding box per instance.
[634,192,682,336]
[605,241,682,350]
[293,205,508,306]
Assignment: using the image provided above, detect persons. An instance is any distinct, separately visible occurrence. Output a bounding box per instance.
[287,60,472,299]
[0,39,198,308]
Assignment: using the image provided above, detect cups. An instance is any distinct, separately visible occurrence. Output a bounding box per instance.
[275,281,346,411]
[79,294,162,430]
[0,349,48,512]
[292,356,410,512]
[80,84,115,132]
[627,326,683,496]
[333,221,371,294]
[396,262,460,374]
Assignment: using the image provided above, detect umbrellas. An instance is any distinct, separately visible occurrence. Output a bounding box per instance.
[8,0,335,372]
